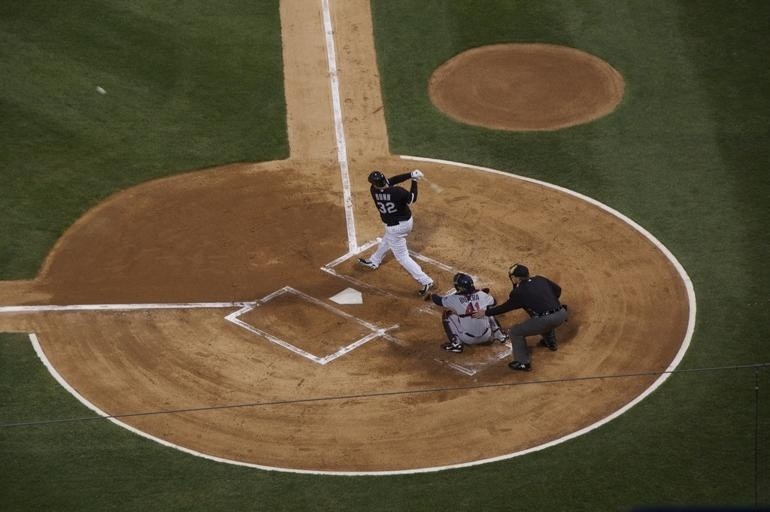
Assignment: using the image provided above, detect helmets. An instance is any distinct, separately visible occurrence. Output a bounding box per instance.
[366,169,388,188]
[454,273,474,288]
[508,264,530,278]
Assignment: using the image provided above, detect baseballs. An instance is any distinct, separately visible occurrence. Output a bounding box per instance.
[96,86,106,95]
[416,173,444,193]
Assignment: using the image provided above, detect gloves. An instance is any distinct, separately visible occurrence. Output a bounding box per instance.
[410,168,425,182]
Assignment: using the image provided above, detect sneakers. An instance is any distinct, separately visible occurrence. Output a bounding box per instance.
[439,342,464,353]
[356,256,379,271]
[417,280,435,296]
[508,360,533,371]
[540,338,559,351]
[495,334,509,344]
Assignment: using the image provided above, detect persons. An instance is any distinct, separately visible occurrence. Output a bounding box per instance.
[356,170,434,295]
[430,272,509,355]
[472,263,569,371]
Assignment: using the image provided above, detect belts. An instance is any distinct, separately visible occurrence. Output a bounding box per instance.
[464,327,490,339]
[386,221,400,227]
[537,305,564,317]
